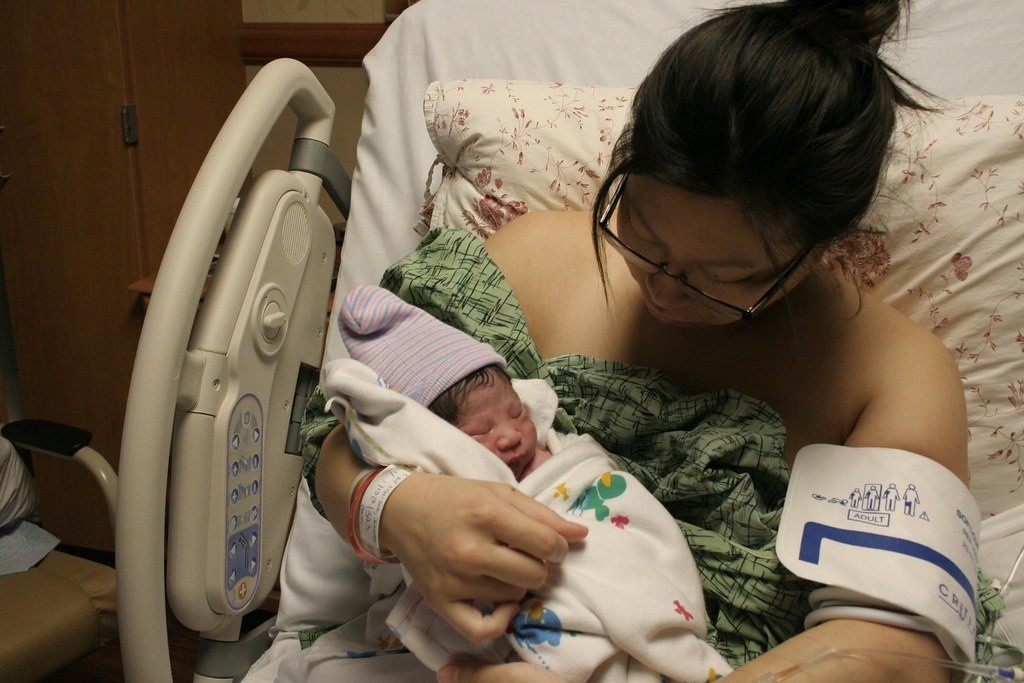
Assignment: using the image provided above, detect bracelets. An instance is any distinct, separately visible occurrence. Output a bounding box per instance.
[345,465,403,565]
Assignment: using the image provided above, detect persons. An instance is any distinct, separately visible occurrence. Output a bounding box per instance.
[312,1,979,681]
[325,283,735,683]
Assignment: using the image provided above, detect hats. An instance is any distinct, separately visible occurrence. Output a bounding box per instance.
[339,284,506,409]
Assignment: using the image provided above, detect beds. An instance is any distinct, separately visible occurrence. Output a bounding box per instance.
[109,1,1024,683]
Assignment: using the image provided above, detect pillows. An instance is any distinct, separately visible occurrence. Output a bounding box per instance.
[413,75,1024,522]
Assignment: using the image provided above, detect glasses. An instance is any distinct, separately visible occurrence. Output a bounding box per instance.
[598,172,827,325]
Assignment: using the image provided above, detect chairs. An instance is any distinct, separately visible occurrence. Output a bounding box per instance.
[0,417,118,683]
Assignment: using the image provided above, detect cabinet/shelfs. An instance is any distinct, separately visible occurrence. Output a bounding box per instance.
[0,0,249,559]
